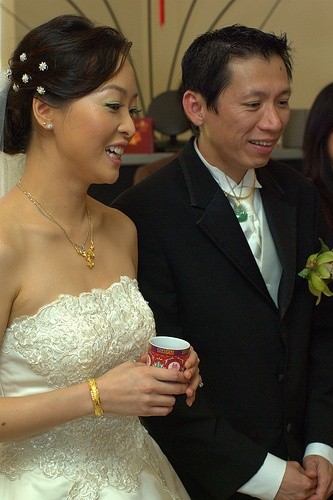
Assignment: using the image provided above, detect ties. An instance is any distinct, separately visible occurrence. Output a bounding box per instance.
[229,186,263,260]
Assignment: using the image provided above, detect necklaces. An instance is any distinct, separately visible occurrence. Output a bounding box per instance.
[16,179,96,271]
[221,172,256,222]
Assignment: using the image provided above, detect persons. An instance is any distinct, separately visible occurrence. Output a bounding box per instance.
[0,14,203,500]
[109,24,332,500]
[302,82,332,196]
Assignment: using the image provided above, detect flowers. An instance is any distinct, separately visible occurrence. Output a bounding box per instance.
[298,238,333,305]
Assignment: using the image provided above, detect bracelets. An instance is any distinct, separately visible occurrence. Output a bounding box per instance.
[87,379,104,417]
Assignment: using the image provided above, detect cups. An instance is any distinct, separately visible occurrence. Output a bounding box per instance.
[147,336,191,383]
